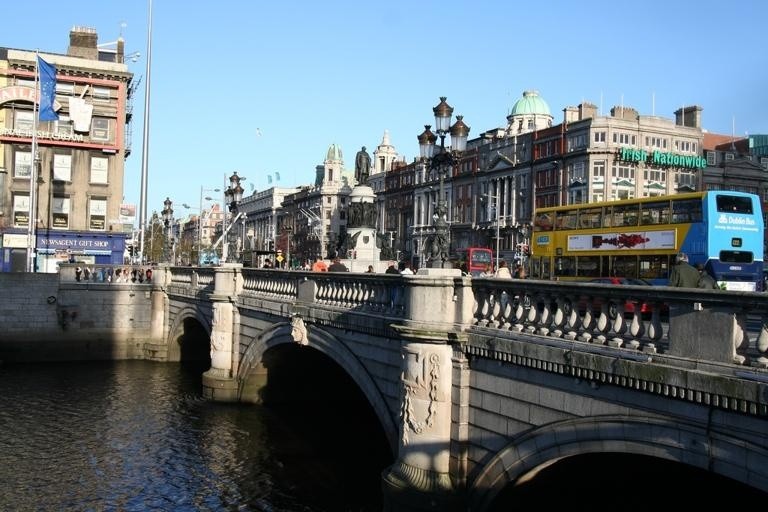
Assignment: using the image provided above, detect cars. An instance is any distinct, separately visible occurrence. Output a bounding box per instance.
[449,252,459,261]
[764,267,768,280]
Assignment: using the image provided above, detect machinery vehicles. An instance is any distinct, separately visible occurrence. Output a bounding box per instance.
[198,212,242,267]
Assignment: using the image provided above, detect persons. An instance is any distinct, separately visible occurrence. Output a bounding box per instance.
[356,146,372,187]
[693,262,720,290]
[302,255,415,310]
[664,251,701,339]
[512,261,526,283]
[495,260,511,277]
[74,261,153,283]
[262,258,275,268]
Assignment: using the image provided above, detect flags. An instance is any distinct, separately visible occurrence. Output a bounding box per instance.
[36,54,60,122]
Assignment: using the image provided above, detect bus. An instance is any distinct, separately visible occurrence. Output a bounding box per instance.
[529,190,763,320]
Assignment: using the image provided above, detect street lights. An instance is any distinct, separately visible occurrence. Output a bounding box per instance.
[138,185,220,262]
[224,171,245,263]
[417,96,471,269]
[491,193,500,270]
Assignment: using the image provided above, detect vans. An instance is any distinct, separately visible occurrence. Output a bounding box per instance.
[455,247,494,274]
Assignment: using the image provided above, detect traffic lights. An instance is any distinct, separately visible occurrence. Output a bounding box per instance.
[269,241,274,252]
[519,232,524,238]
[516,245,521,256]
[134,247,138,256]
[128,246,133,256]
